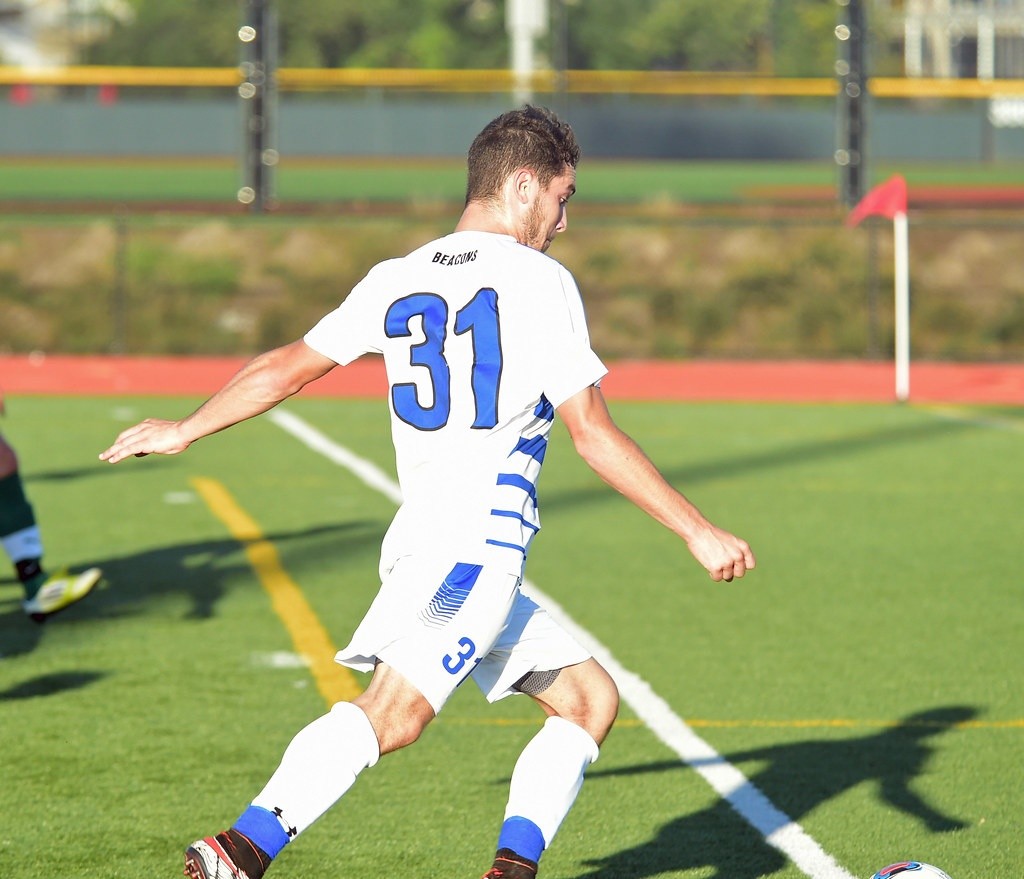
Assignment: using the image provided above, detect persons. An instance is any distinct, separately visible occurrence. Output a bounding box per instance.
[0,433,104,614]
[96,100,757,879]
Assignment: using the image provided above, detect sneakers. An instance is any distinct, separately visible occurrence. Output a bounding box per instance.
[22,565,103,623]
[184,831,250,879]
[483,866,511,879]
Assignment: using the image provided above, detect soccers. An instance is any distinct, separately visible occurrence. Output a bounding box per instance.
[869,861,951,879]
